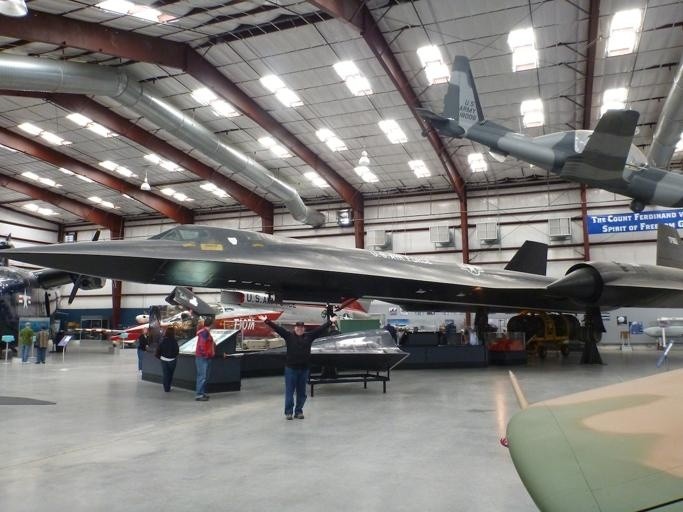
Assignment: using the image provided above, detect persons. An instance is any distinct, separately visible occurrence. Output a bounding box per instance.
[258,314,337,420]
[195,318,216,401]
[137,333,149,370]
[158,327,179,392]
[19,322,50,364]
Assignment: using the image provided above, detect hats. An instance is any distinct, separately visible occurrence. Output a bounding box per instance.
[295,320,304,327]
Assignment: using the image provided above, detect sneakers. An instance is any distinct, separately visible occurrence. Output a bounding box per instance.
[195,394,209,400]
[286,413,305,420]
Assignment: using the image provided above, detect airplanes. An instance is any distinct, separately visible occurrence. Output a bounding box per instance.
[415,55,683,213]
